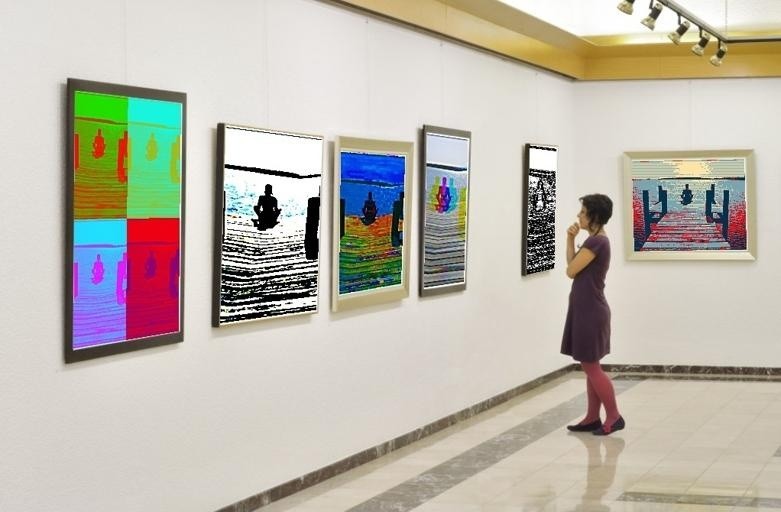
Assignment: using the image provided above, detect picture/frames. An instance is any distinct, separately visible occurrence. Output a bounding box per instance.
[330,134,416,314]
[63,75,189,366]
[621,149,761,262]
[417,124,472,299]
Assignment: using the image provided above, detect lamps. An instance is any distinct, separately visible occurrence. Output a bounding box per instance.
[616,0,730,68]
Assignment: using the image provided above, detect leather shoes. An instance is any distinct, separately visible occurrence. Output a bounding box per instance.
[566,416,603,433]
[593,414,626,436]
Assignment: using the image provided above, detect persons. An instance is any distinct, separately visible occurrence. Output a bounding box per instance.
[558,193,628,437]
